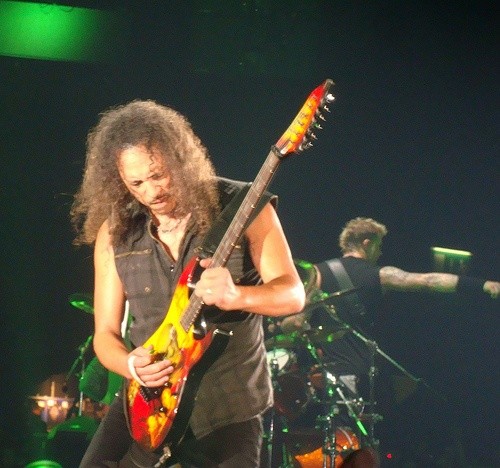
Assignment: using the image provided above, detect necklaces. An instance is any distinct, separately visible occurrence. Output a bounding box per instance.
[155,218,182,232]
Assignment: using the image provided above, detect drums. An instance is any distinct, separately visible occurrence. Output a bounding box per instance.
[292,428,361,468]
[319,400,339,417]
[310,360,357,388]
[335,400,375,425]
[266,349,308,422]
[283,427,326,455]
[341,446,378,468]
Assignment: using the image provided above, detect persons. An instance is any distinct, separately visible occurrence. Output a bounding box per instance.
[74,102,306,468]
[268,217,500,468]
[32,373,108,434]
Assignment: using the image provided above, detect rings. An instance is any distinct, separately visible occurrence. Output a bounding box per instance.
[207,288,213,294]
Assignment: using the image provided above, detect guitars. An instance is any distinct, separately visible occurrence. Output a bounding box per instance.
[121,77,337,453]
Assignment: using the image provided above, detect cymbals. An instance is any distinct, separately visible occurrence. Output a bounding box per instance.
[357,414,382,422]
[26,395,73,401]
[68,293,94,314]
[264,326,351,348]
[294,257,321,299]
[266,287,360,322]
